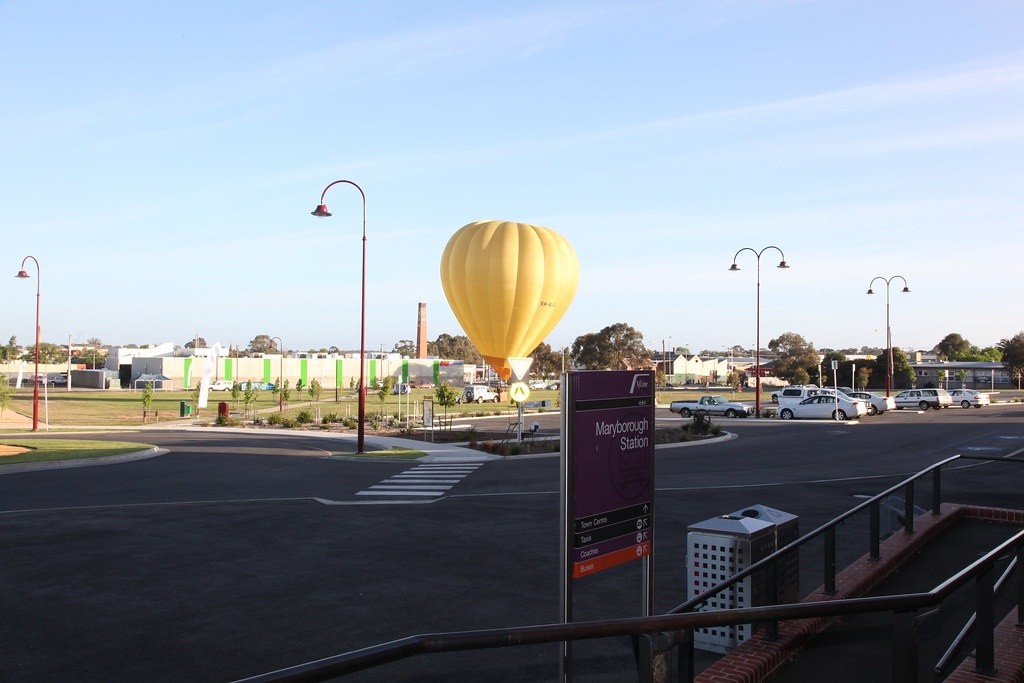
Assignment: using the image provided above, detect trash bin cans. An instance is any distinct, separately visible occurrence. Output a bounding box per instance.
[684,503,801,657]
[180,400,187,418]
[186,406,192,413]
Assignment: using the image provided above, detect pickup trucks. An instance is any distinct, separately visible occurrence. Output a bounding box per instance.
[892,388,954,411]
[778,387,871,418]
[47,377,69,385]
[669,394,756,419]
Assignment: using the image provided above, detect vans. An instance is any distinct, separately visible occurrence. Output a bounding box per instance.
[394,384,413,394]
[60,374,68,383]
[31,373,45,385]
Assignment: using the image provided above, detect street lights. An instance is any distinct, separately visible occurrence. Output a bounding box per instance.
[731,246,790,417]
[266,335,284,415]
[866,275,911,398]
[15,255,42,435]
[308,178,366,457]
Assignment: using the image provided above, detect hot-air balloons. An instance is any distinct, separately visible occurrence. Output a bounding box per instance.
[438,221,582,403]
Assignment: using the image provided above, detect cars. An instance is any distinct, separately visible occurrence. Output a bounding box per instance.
[777,395,867,421]
[415,381,436,389]
[402,380,418,389]
[845,392,896,417]
[528,381,547,390]
[943,388,992,410]
[548,382,557,390]
[266,383,277,390]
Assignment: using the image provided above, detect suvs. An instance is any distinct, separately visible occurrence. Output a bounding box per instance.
[209,381,233,392]
[463,385,501,404]
[771,386,789,404]
